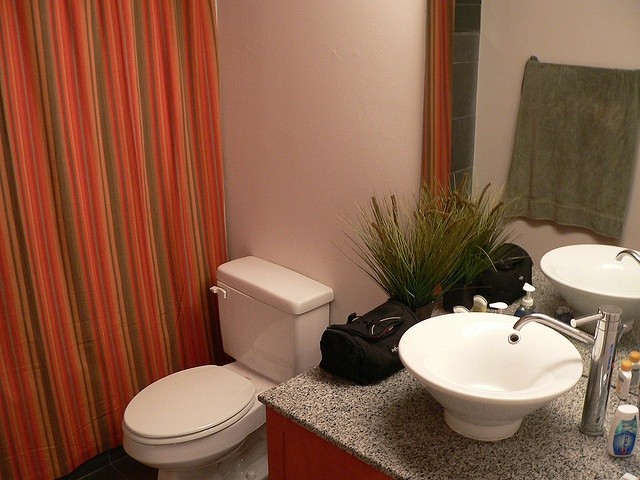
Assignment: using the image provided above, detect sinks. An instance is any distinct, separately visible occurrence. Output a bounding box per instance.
[540,244,639,331]
[399,313,583,443]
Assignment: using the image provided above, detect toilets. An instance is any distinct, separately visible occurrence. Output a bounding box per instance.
[121,255,334,476]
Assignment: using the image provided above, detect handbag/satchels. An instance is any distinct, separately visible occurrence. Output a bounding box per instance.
[317,297,421,386]
[442,242,534,313]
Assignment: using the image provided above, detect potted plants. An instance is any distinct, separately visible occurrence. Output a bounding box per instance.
[330,192,484,323]
[415,174,521,311]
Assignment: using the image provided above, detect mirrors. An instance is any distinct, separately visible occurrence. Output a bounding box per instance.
[422,2,639,397]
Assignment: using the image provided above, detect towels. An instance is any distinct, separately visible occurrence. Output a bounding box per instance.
[498,56,639,239]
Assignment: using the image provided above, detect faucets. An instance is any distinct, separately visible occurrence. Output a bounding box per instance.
[514,304,623,438]
[614,250,640,268]
